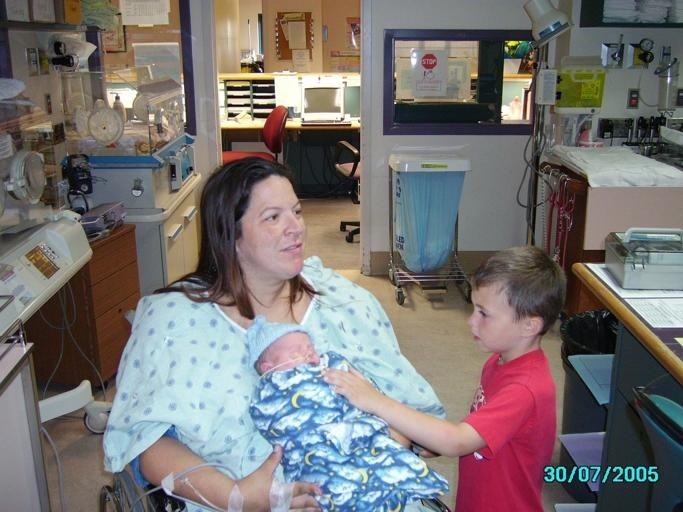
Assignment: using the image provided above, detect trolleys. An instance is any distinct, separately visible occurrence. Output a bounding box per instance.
[381,154,478,313]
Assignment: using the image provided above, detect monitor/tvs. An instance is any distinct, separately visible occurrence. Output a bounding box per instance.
[302,76,345,121]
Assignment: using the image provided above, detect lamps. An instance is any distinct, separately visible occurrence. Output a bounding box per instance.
[522,0,573,48]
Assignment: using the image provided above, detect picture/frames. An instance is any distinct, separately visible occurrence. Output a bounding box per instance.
[100,13,126,53]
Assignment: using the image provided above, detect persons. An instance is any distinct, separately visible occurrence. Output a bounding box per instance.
[241,311,448,510]
[320,243,568,512]
[101,155,449,511]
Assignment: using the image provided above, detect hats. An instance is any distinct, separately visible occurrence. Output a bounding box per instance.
[244,315,312,370]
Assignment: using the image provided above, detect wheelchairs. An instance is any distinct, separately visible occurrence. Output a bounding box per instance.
[92,307,192,512]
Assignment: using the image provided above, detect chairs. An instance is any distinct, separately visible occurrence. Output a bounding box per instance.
[333,142,360,242]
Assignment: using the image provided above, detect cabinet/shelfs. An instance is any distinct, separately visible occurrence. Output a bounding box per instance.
[219,70,361,200]
[25,221,141,389]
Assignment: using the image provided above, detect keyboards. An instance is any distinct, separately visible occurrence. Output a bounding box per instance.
[301,120,352,126]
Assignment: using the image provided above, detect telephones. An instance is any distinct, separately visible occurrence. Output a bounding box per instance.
[288,106,300,118]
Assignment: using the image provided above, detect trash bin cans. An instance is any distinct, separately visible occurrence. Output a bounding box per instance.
[388,153,471,274]
[560,308,620,504]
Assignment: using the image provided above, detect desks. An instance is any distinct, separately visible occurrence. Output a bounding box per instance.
[0,338,52,511]
[571,263,683,512]
[544,146,683,320]
